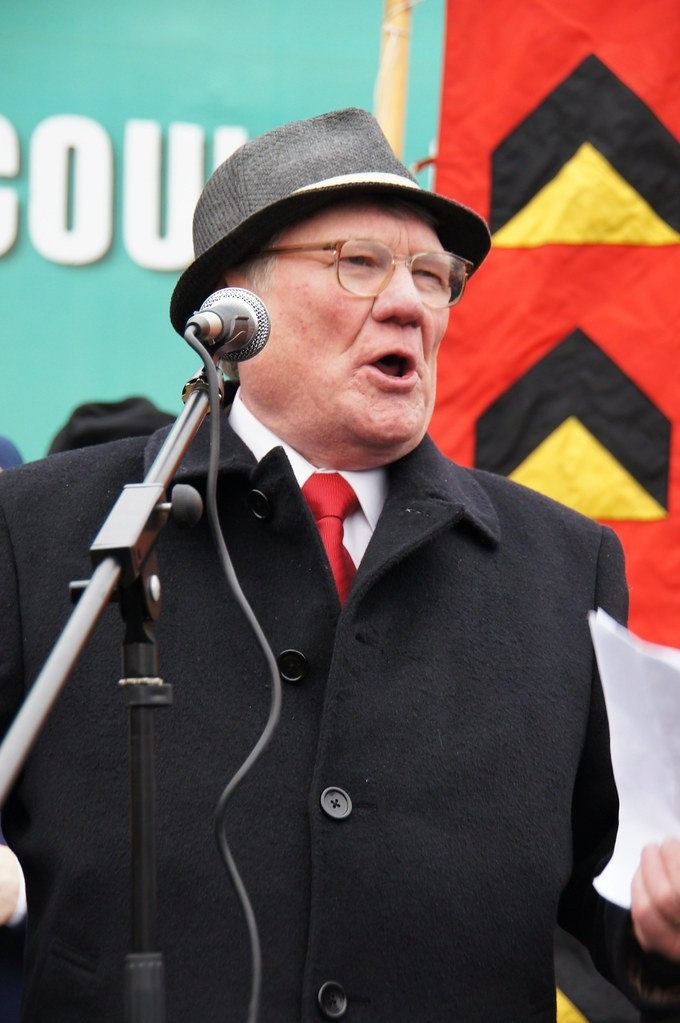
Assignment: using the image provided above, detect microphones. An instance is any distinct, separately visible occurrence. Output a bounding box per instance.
[181,287,270,362]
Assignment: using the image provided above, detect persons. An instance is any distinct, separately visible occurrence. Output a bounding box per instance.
[0,108,679,1023]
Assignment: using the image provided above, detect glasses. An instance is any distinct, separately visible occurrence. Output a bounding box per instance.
[234,241,477,310]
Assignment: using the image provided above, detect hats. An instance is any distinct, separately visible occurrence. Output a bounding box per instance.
[168,109,491,348]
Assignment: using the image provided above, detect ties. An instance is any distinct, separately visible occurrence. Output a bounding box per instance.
[303,474,359,606]
